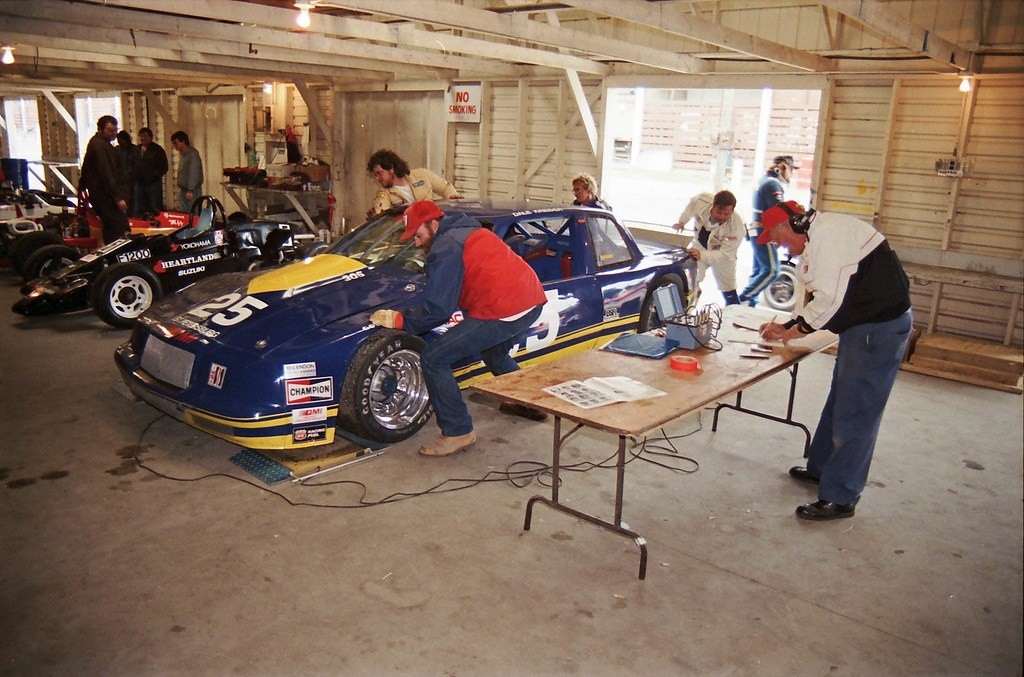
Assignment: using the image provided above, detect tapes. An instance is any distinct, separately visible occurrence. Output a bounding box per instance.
[670,355,702,375]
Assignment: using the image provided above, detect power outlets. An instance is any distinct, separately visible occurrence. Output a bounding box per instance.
[947,160,964,178]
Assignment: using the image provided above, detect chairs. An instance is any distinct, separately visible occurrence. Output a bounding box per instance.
[179,207,214,238]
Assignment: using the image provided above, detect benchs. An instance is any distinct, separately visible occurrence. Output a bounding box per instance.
[503,236,590,280]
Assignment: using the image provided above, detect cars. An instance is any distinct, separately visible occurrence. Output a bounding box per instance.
[113,197,704,452]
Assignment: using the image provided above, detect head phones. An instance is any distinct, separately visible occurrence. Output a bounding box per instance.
[777,203,811,234]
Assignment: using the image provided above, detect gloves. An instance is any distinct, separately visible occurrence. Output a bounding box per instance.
[370,309,403,330]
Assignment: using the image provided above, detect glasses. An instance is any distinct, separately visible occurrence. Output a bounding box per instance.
[373,169,386,177]
[571,188,586,195]
[105,126,118,132]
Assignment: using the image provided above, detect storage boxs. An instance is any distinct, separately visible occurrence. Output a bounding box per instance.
[225,159,331,189]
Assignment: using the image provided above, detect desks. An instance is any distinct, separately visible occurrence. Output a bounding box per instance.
[468,303,841,581]
[219,182,330,237]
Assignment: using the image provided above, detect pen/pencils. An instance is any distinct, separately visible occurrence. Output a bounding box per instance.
[740,355,769,359]
[759,314,778,339]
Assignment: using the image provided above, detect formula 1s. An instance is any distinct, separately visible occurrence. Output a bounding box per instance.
[9,190,334,334]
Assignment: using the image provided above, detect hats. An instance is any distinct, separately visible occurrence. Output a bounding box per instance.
[755,201,804,244]
[774,156,800,169]
[400,201,444,241]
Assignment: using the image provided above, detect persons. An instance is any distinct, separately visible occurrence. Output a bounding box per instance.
[571,173,613,213]
[114,130,137,207]
[370,202,549,457]
[365,149,465,222]
[672,190,745,307]
[171,130,205,214]
[739,155,800,308]
[756,200,913,521]
[131,126,169,220]
[79,116,131,246]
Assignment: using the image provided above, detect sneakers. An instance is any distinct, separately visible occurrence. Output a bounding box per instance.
[418,431,476,457]
[789,465,820,484]
[498,401,551,423]
[796,498,855,521]
